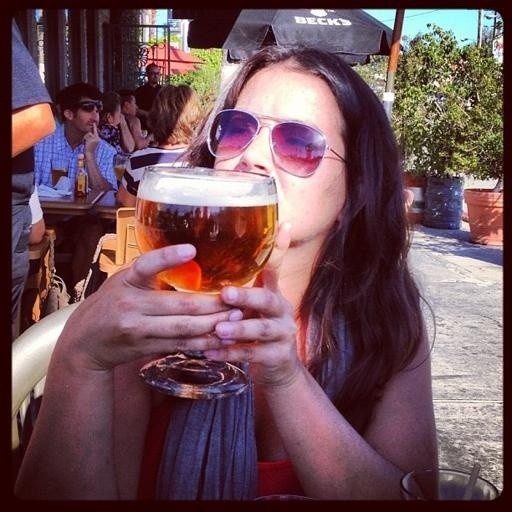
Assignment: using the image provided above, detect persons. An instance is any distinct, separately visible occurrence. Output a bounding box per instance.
[17,61,205,333]
[11,20,56,344]
[10,38,442,507]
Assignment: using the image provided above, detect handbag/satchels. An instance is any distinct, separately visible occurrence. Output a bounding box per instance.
[41,275,70,319]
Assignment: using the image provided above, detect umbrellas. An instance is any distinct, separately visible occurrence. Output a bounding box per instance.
[184,2,403,70]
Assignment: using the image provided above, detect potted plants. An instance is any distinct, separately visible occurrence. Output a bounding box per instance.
[390,23,504,246]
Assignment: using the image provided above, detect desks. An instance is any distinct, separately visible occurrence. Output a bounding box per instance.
[92,190,116,220]
[38,190,104,264]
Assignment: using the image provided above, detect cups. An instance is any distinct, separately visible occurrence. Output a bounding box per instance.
[397,467,500,504]
[50,157,70,190]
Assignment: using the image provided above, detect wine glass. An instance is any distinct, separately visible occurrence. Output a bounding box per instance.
[112,153,129,182]
[131,164,281,402]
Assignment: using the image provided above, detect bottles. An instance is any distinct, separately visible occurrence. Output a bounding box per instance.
[72,153,90,198]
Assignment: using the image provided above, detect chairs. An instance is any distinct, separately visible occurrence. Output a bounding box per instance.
[21,229,56,329]
[99,207,141,282]
[11,299,85,482]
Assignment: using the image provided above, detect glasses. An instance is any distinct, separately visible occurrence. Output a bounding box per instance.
[75,100,104,112]
[206,109,348,178]
